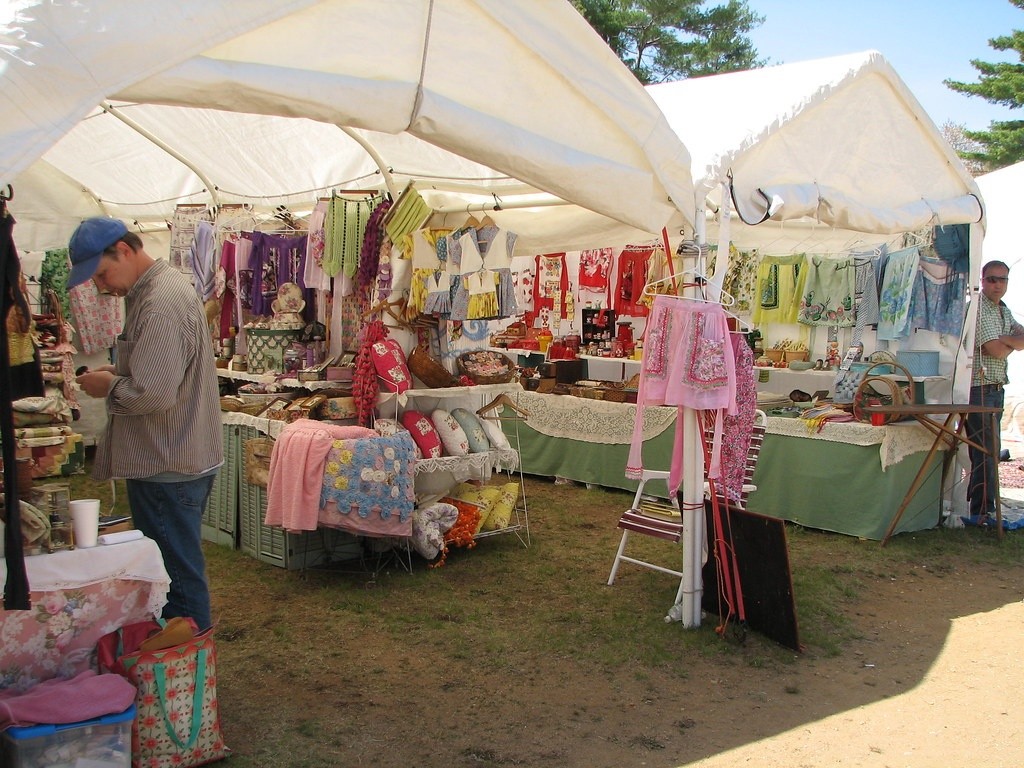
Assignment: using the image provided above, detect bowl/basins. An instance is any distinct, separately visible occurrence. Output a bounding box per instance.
[786,351,808,364]
[765,350,786,362]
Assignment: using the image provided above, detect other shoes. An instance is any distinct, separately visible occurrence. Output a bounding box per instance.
[988,511,1006,520]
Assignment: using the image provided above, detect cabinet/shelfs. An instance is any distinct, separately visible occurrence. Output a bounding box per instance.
[357,383,529,575]
[32,486,74,553]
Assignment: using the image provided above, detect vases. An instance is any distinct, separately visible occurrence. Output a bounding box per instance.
[785,351,807,364]
[764,348,783,360]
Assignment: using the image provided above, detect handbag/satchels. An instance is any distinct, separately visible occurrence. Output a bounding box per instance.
[94,616,232,767]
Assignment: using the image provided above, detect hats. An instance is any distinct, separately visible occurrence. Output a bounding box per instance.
[65,216,129,290]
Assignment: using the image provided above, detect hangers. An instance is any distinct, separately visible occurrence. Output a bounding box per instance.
[616,214,942,334]
[177,171,503,335]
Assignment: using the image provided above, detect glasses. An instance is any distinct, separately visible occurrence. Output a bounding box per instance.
[982,276,1010,283]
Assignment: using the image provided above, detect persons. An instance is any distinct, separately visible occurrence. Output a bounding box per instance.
[67,219,224,636]
[958,260,1024,521]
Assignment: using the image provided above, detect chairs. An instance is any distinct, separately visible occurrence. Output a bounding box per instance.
[607,408,766,603]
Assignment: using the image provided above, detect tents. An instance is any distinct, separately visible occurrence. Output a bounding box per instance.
[0,0,987,627]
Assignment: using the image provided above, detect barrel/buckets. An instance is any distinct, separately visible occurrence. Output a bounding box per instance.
[538,337,551,352]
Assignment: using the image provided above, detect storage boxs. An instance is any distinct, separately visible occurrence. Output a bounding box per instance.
[895,350,940,378]
[4,702,142,767]
[325,367,353,380]
[296,371,324,380]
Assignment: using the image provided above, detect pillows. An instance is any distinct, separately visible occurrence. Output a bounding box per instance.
[430,404,468,455]
[478,412,512,452]
[449,483,497,534]
[372,417,423,458]
[371,407,512,466]
[450,407,493,453]
[373,339,412,394]
[481,481,519,531]
[400,408,442,458]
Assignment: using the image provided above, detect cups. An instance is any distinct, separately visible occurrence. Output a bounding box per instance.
[871,405,885,426]
[69,499,99,548]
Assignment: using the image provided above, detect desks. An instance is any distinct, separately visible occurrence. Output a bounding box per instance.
[863,404,1008,548]
[1,536,174,695]
[503,391,948,541]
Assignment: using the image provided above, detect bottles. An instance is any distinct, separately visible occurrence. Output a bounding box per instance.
[580,336,623,357]
[567,329,581,354]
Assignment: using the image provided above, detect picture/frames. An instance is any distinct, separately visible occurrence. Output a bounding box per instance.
[333,349,358,367]
[255,397,292,419]
[285,397,313,414]
[301,394,327,409]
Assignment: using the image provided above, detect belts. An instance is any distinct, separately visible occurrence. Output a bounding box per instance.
[971,384,998,392]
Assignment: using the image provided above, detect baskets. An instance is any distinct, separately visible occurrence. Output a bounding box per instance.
[457,349,516,383]
[408,347,455,388]
